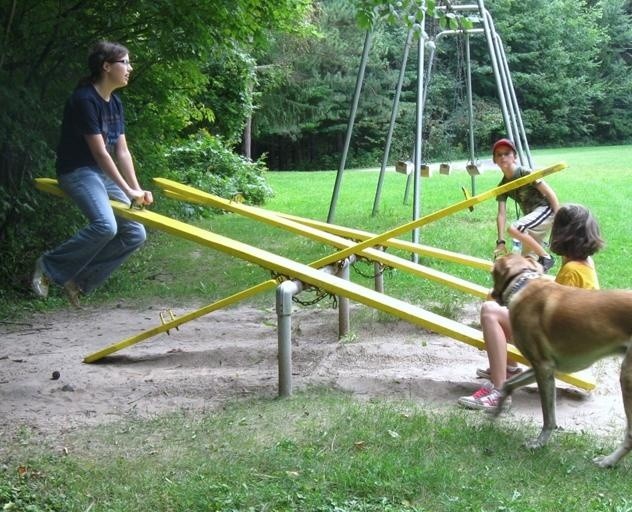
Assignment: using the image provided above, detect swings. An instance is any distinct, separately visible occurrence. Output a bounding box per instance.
[396,20,479,177]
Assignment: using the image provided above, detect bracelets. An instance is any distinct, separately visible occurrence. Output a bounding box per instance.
[496,239,505,244]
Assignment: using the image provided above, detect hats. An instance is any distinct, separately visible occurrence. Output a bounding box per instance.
[492,139,516,155]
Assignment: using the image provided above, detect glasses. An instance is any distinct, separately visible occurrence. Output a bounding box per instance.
[112,59,129,65]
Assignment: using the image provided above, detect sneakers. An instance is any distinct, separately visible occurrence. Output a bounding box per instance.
[32,257,49,299]
[538,254,555,271]
[64,280,81,307]
[458,381,512,411]
[477,364,523,380]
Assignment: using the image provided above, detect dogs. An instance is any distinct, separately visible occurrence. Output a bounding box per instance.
[490,253,632,470]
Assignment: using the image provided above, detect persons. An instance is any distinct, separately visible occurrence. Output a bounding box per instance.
[456,205,604,413]
[492,138,559,273]
[32,40,153,306]
